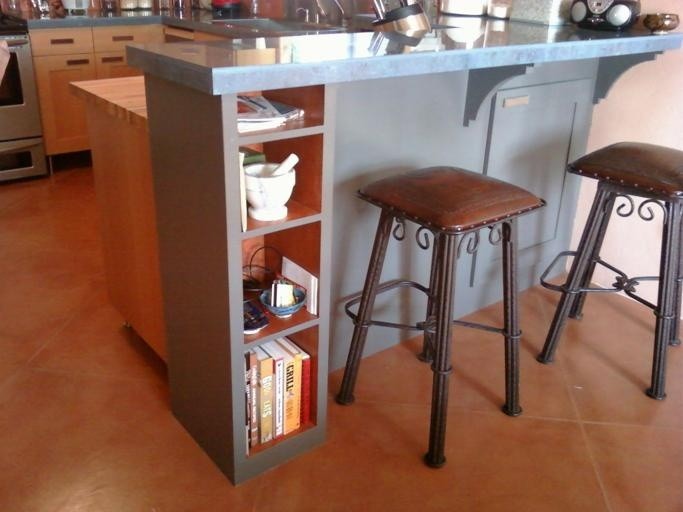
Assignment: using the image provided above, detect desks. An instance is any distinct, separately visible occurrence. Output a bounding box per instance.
[125,27,682,487]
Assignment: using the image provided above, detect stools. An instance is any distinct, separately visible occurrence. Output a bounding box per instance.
[335,166,547,467]
[537,141,682,400]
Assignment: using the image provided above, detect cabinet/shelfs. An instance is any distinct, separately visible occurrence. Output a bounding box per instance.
[68,75,167,364]
[144,75,338,487]
[28,24,165,176]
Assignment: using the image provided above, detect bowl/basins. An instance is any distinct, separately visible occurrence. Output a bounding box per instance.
[72,9,85,15]
[260,288,307,316]
[642,13,678,35]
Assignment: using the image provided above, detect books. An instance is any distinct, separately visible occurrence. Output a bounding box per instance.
[236,146,267,166]
[235,92,309,135]
[240,335,311,450]
[237,152,247,232]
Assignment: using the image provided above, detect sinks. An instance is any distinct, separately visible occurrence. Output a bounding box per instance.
[211,16,346,35]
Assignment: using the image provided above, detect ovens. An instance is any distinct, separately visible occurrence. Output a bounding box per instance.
[0,31,49,181]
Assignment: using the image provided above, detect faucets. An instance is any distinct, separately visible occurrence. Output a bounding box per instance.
[331,0,347,28]
[314,0,326,19]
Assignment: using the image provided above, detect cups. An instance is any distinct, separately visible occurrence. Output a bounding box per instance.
[487,0,512,19]
